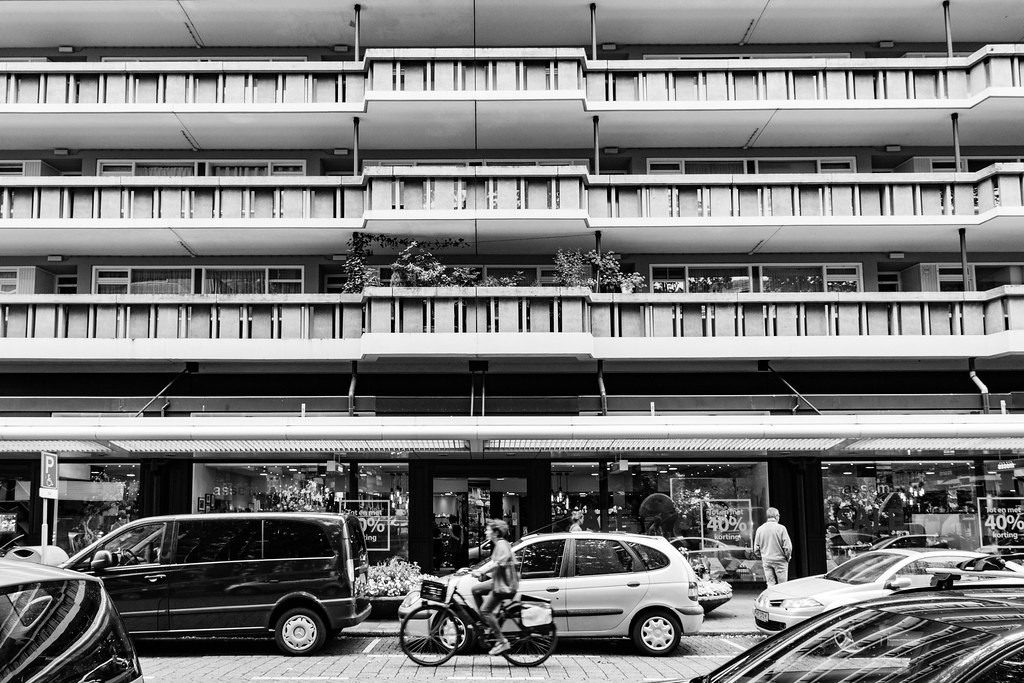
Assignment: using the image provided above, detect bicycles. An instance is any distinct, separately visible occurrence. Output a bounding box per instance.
[400,567,559,670]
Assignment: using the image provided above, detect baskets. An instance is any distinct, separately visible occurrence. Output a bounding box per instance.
[419,580,447,602]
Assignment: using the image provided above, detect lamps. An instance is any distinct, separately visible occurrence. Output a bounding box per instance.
[895,471,925,505]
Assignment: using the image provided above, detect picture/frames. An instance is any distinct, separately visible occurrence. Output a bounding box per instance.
[198,493,231,514]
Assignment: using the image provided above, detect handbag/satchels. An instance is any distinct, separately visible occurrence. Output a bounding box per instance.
[493,564,517,595]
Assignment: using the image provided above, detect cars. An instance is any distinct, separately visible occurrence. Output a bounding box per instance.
[398,530,704,657]
[0,561,145,683]
[754,545,1024,634]
[683,578,1023,682]
[866,532,955,552]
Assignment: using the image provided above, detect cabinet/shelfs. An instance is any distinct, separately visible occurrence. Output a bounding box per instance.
[434,491,469,571]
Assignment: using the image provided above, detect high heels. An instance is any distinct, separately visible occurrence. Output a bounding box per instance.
[489,638,511,655]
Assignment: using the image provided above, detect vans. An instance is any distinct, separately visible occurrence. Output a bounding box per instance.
[59,511,372,656]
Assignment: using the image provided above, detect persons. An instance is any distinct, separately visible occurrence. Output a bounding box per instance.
[648,516,665,535]
[447,514,466,570]
[471,519,518,655]
[752,509,791,590]
[433,511,445,572]
[569,512,584,532]
